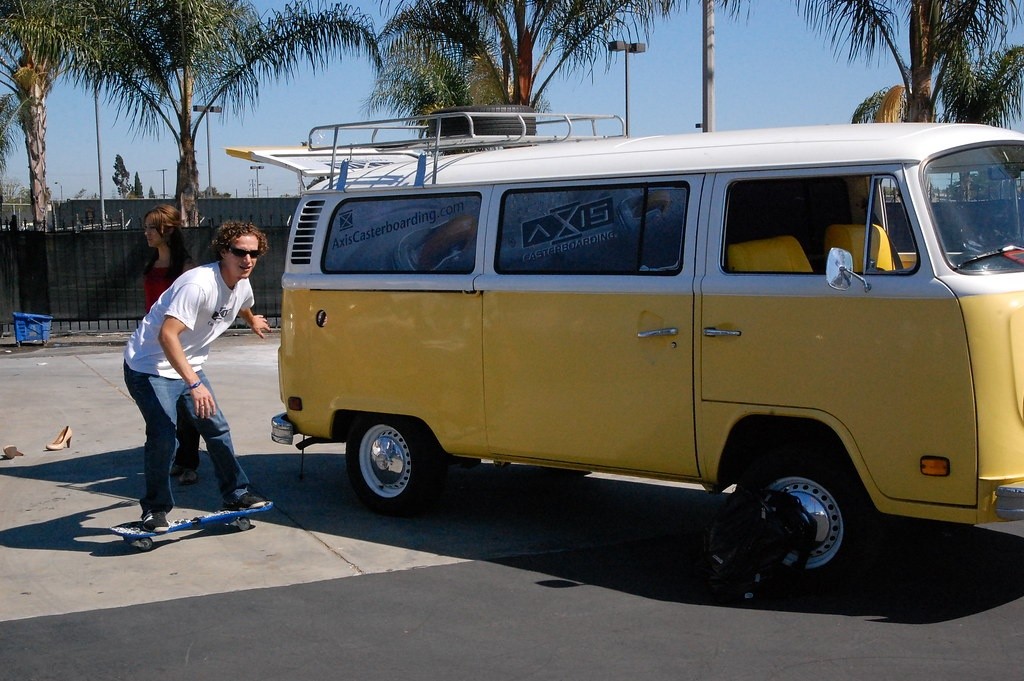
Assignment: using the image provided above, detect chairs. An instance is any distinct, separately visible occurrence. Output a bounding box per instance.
[727,235,813,273]
[824,222,896,271]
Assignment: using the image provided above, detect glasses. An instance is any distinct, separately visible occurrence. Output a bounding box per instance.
[227,246,259,259]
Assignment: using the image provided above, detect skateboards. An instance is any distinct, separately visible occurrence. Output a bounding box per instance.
[109,501,274,552]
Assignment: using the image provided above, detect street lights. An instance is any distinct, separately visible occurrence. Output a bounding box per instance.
[607,40,646,140]
[193,103,223,198]
[156,168,168,199]
[54,181,63,201]
[250,165,265,199]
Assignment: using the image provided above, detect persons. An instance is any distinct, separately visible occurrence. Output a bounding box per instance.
[122,221,273,533]
[141,203,200,484]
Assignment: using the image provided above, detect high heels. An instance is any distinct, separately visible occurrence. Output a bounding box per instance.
[46,425,72,450]
[3,445,23,459]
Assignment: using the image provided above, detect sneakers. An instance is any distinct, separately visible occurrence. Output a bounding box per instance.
[141,510,169,532]
[218,493,265,515]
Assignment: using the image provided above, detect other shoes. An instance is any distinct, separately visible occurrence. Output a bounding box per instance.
[170,458,199,486]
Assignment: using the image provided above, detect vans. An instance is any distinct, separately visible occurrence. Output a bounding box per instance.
[223,106,1023,581]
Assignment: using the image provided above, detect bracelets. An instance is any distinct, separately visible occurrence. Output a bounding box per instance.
[189,380,202,389]
